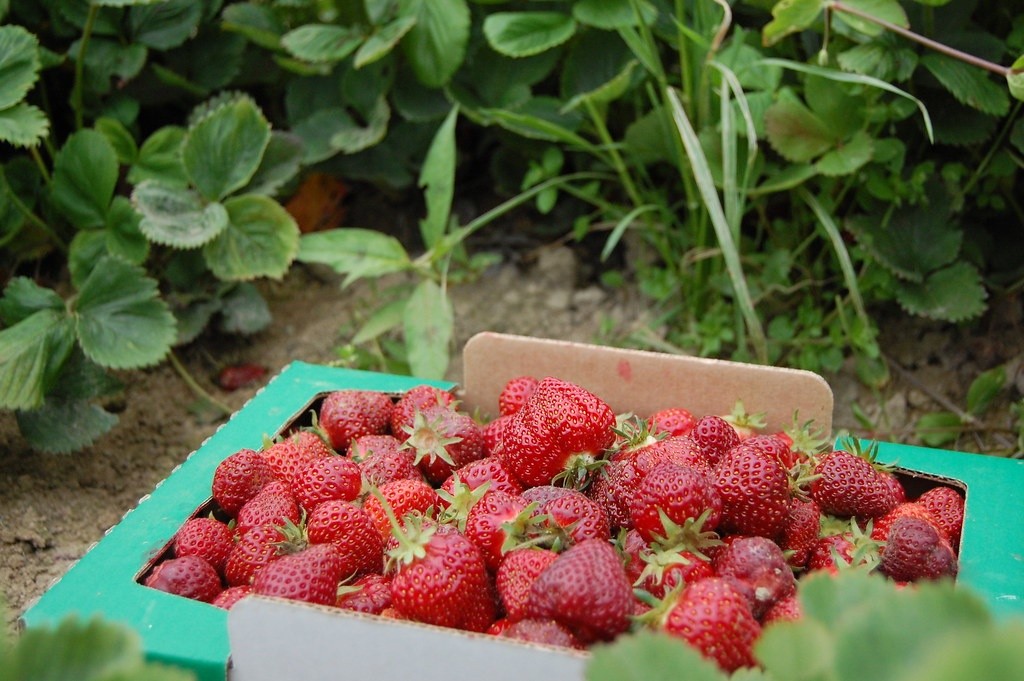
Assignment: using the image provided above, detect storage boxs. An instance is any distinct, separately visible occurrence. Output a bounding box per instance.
[16,333,1024,681]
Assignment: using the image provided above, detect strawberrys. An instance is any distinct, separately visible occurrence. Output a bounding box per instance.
[141,377,964,671]
